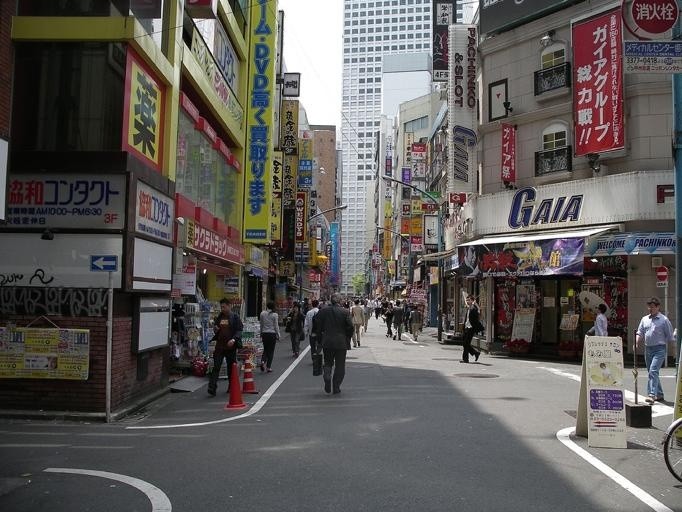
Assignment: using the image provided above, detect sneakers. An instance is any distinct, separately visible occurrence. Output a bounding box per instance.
[386,329,417,341]
[267,368,272,372]
[654,394,663,400]
[260,361,265,371]
[645,396,654,405]
[208,387,216,396]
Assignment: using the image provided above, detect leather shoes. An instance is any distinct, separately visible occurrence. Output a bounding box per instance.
[293,351,296,357]
[460,360,468,362]
[475,352,480,361]
[296,351,299,357]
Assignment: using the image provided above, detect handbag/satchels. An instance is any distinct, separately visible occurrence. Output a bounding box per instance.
[312,353,322,376]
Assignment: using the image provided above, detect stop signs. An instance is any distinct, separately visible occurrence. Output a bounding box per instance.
[656,265,669,282]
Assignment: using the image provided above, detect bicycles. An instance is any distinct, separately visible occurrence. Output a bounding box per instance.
[660,418,682,483]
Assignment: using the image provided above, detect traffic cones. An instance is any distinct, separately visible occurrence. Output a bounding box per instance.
[240,354,259,394]
[224,363,248,411]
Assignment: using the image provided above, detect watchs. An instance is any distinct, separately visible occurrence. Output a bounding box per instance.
[232,339,236,342]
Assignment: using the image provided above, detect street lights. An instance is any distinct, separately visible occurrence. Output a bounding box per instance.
[382,175,443,342]
[376,225,412,284]
[299,204,347,303]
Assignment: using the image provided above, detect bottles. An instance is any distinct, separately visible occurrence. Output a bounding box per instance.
[241,316,265,365]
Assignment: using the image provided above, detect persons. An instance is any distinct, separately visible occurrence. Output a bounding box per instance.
[459,245,477,275]
[315,294,354,395]
[208,299,243,396]
[633,297,676,405]
[284,296,421,358]
[587,303,608,336]
[260,300,280,372]
[460,295,484,363]
